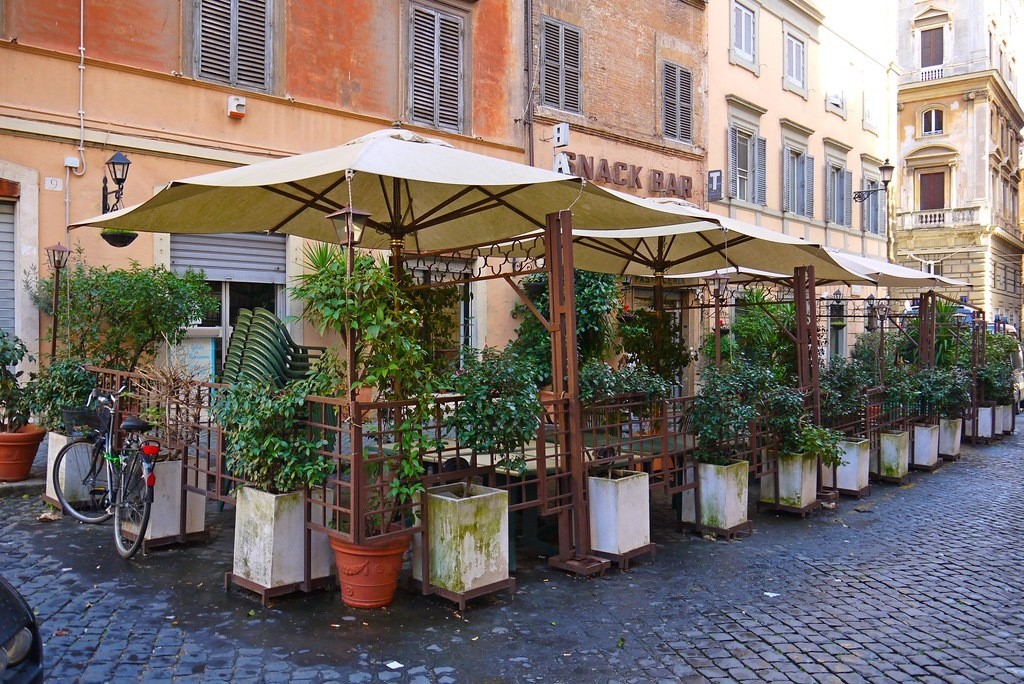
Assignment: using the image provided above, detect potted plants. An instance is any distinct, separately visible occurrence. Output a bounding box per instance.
[563,267,1023,556]
[101,228,138,248]
[277,241,552,608]
[208,345,348,588]
[0,235,222,540]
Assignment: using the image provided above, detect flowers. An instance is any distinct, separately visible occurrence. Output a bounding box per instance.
[719,320,729,329]
[621,305,634,317]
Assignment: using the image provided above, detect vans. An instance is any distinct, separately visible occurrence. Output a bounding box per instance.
[982,324,1024,415]
[897,302,974,381]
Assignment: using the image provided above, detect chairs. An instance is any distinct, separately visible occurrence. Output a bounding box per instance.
[218,307,338,460]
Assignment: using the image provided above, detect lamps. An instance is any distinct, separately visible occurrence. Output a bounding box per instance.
[703,270,731,298]
[826,289,844,308]
[853,159,895,202]
[325,203,373,247]
[102,150,132,214]
[952,313,968,327]
[44,242,73,268]
[867,293,875,310]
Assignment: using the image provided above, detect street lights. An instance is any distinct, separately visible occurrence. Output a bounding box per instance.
[323,205,372,402]
[703,271,731,373]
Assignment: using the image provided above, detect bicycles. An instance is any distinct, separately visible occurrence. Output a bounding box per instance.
[53,381,161,560]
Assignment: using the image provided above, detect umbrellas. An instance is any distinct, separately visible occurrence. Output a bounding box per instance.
[451,187,972,376]
[66,117,723,313]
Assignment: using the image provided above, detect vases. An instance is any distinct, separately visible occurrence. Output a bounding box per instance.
[713,329,729,335]
[617,318,633,323]
